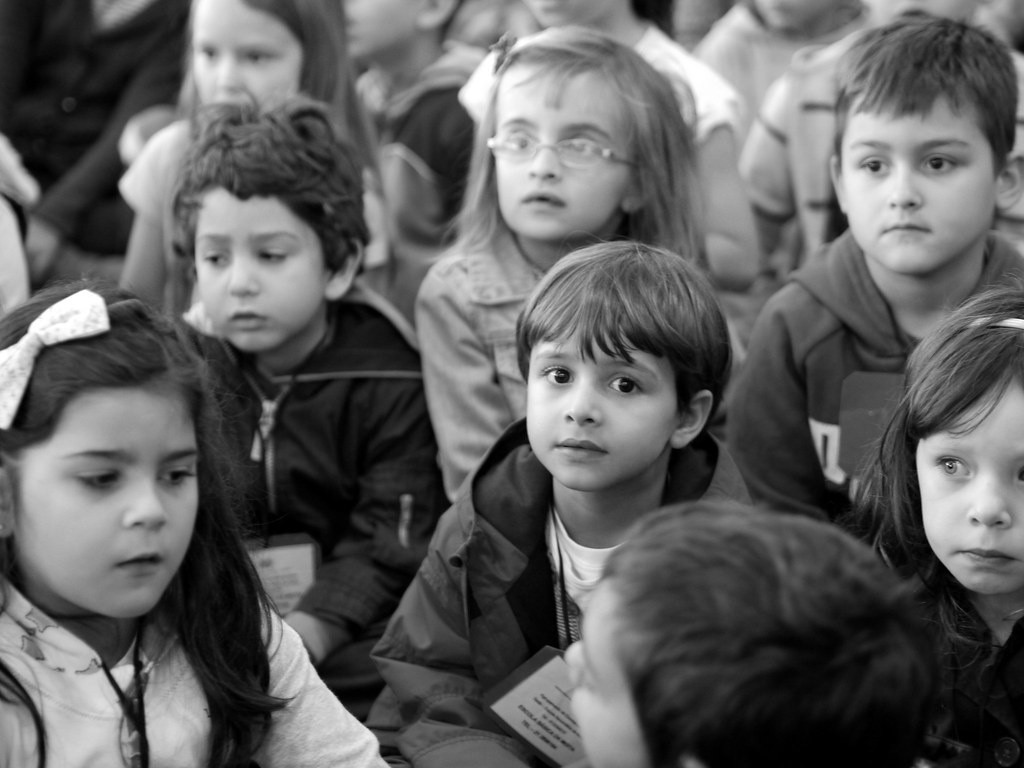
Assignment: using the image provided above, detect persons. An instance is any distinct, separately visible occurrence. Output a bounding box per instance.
[413,29,709,517]
[366,242,758,768]
[690,0,1022,316]
[566,501,936,768]
[852,289,1023,768]
[458,0,758,282]
[735,17,1023,518]
[0,0,489,767]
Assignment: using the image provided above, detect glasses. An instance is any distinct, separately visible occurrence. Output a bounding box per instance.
[486,135,639,170]
